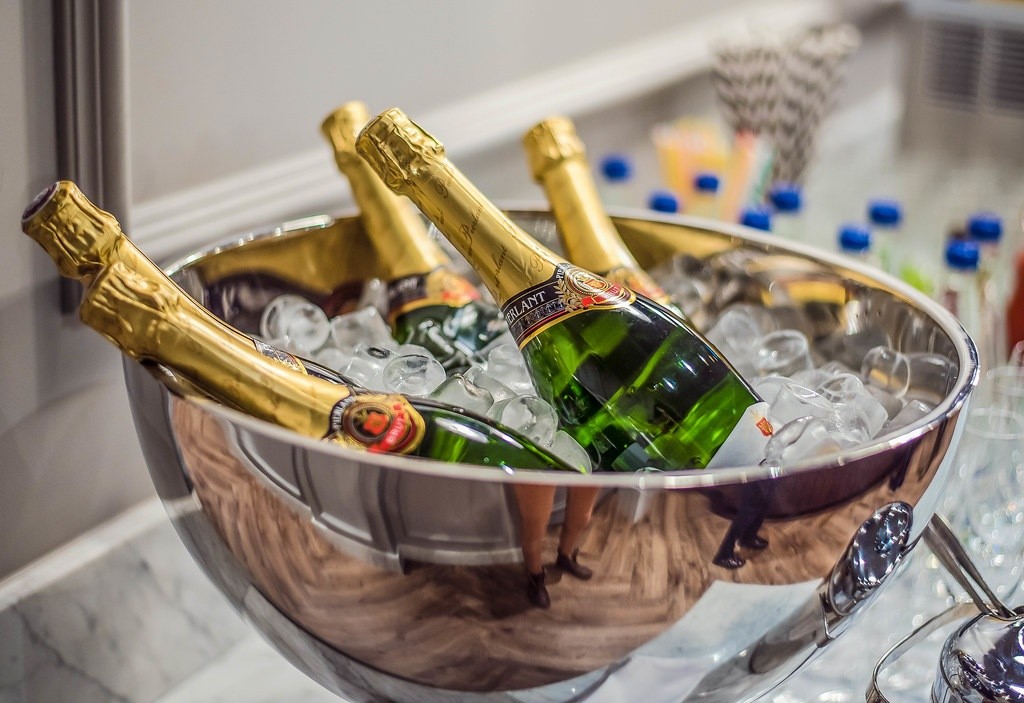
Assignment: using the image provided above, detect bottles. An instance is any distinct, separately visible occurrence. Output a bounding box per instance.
[321,97,505,377]
[353,107,772,473]
[521,116,695,333]
[20,179,362,389]
[600,155,1002,366]
[79,260,579,474]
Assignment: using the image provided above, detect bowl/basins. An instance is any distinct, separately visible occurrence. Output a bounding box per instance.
[137,205,981,703]
